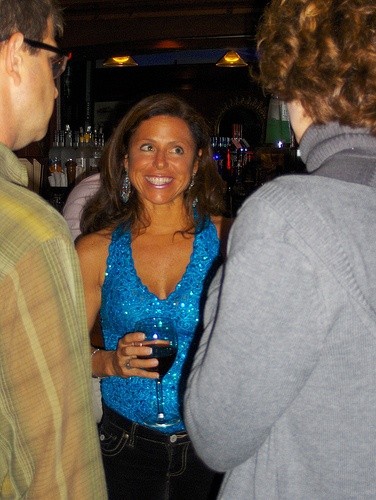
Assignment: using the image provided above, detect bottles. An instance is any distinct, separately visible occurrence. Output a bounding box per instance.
[208,137,253,176]
[50,157,99,187]
[52,126,105,146]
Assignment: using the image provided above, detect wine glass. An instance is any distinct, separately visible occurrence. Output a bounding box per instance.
[133,317,180,428]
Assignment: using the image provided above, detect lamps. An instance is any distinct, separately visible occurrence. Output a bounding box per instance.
[102,55,138,67]
[216,50,248,67]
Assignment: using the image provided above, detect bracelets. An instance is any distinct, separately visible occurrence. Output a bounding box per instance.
[89,348,100,379]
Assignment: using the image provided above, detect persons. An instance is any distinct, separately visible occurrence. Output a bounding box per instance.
[73,96,227,499]
[180,0,375,500]
[62,172,132,425]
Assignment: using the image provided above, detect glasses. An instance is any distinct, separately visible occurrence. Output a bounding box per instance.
[0,36,69,80]
